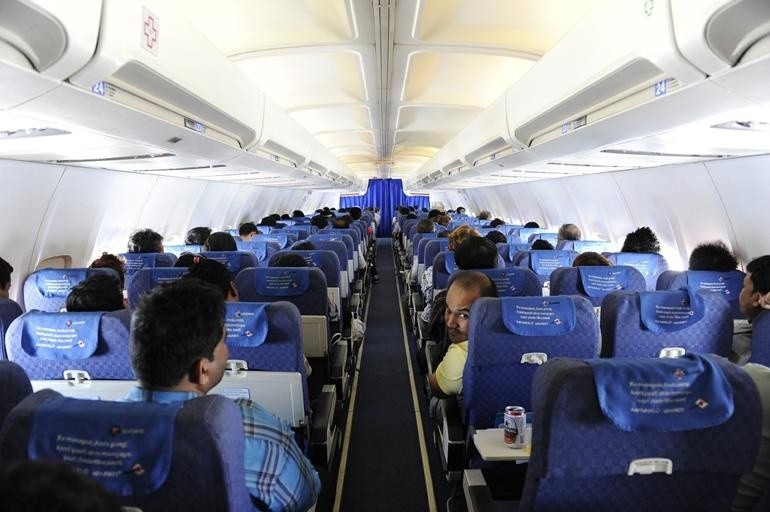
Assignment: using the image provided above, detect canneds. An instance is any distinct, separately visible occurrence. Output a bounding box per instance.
[504,405,527,448]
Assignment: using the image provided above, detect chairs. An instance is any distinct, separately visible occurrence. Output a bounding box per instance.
[0,205,383,511]
[391,205,770,510]
